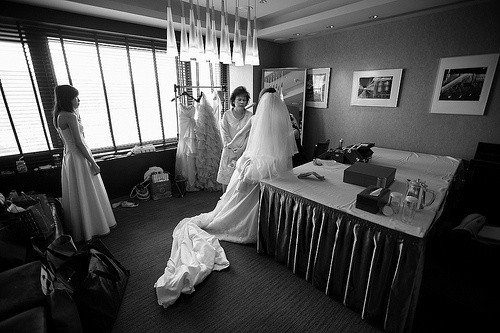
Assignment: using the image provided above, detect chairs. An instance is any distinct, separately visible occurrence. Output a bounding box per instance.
[0,261,49,333]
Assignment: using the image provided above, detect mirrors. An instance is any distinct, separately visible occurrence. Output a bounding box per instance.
[261,68,306,146]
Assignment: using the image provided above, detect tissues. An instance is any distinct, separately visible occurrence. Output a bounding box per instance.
[355,184,391,214]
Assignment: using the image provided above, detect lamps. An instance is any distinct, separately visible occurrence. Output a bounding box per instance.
[268,73,284,101]
[164,0,260,66]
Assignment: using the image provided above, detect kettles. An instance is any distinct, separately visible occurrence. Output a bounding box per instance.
[406,178,437,211]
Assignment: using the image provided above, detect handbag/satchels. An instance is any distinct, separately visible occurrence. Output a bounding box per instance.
[4,192,130,333]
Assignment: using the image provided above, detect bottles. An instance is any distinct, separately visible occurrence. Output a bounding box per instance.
[402,196,418,225]
[390,193,403,214]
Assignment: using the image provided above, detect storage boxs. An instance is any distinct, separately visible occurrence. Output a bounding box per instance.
[343,162,396,189]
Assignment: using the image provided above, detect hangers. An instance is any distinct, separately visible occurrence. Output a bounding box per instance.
[198,89,203,102]
[171,90,198,102]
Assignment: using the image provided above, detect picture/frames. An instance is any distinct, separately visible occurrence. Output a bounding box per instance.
[429,52,500,117]
[350,68,403,108]
[305,68,330,109]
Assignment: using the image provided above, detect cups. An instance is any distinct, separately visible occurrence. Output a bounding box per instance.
[377,176,386,189]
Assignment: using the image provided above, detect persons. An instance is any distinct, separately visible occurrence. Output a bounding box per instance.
[216,86,254,192]
[289,113,309,168]
[53,86,117,242]
[224,87,299,245]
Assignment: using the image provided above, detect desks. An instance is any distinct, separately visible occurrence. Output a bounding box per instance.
[256,146,462,333]
